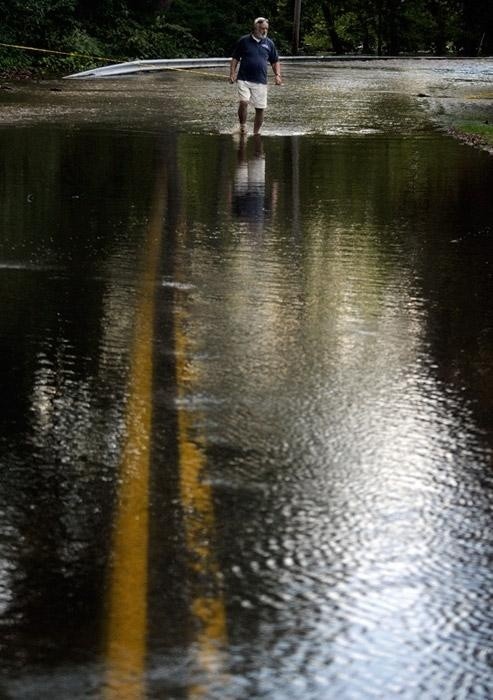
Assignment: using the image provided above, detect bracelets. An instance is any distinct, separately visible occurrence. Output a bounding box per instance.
[276,74,281,77]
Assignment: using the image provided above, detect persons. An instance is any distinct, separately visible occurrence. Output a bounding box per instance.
[226,133,278,239]
[229,18,281,133]
